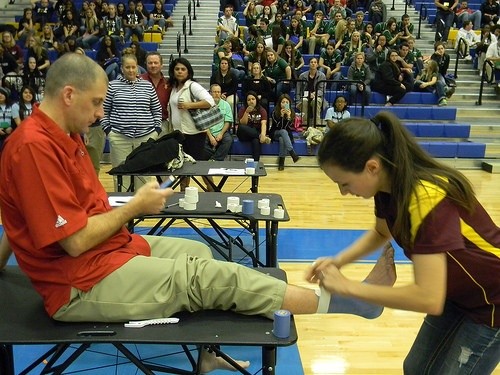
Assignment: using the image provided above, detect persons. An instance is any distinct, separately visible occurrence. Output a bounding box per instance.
[167,57,216,190]
[0,86,13,148]
[13,86,36,130]
[203,84,233,160]
[0,0,173,112]
[324,96,349,134]
[0,52,396,320]
[210,0,500,130]
[138,52,172,141]
[305,110,500,375]
[271,93,302,170]
[100,53,163,192]
[237,90,271,162]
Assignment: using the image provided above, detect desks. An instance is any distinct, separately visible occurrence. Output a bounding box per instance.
[112,161,267,193]
[0,265,298,375]
[106,191,290,267]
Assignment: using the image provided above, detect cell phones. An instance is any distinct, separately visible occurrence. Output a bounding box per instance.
[158,175,176,188]
[285,103,289,110]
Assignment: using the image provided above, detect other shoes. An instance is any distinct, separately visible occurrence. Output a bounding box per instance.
[438,97,448,106]
[259,134,271,145]
[444,86,455,99]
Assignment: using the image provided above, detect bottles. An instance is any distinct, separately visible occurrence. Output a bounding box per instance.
[453,23,456,30]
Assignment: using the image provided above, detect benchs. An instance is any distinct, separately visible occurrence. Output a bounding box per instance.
[0,0,500,170]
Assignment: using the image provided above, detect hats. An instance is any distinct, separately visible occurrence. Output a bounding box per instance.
[0,86,11,102]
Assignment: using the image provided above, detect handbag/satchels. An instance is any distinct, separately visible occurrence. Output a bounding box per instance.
[188,82,225,131]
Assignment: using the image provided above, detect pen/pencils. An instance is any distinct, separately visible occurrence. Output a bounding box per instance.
[77,330,116,336]
[165,202,179,209]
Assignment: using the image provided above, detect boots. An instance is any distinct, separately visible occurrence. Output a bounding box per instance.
[279,157,285,170]
[289,150,299,163]
